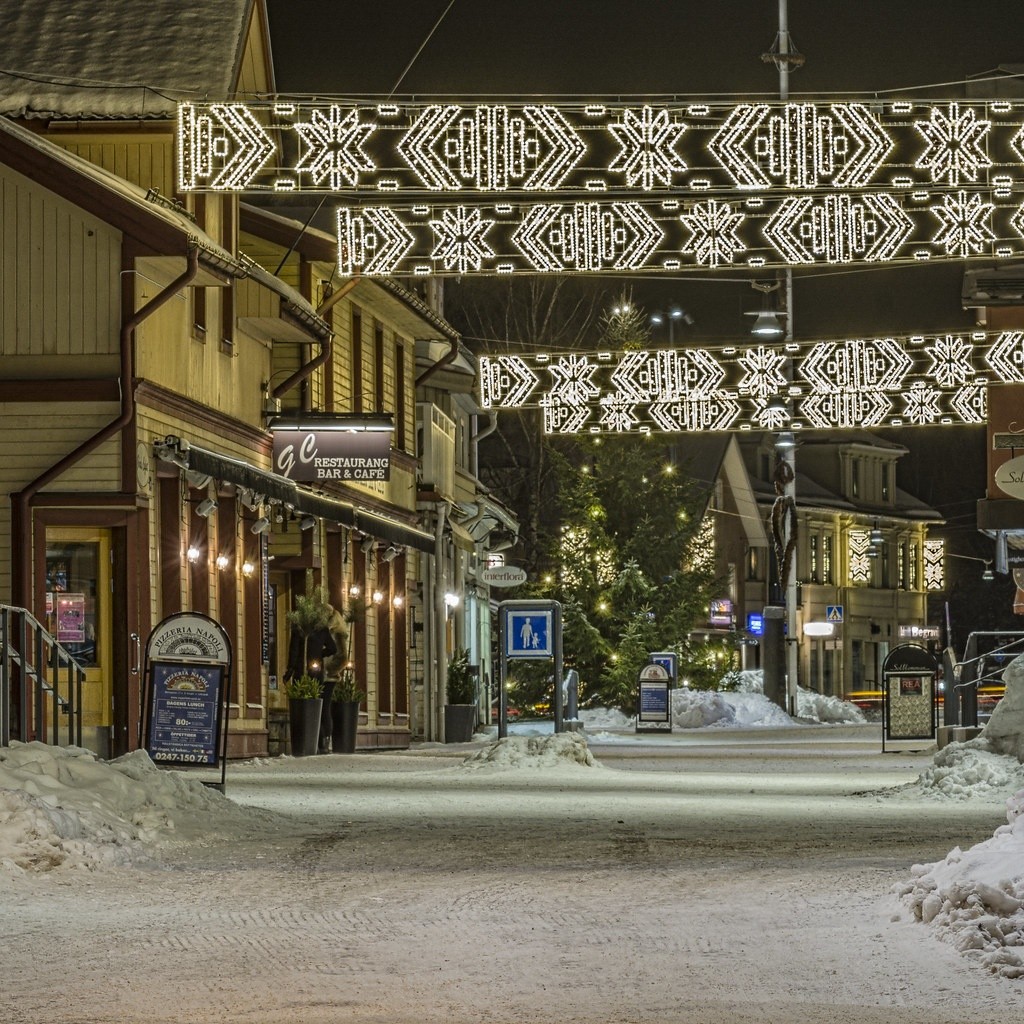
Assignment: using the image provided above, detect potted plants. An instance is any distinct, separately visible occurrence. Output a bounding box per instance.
[331,668,377,754]
[445,645,476,744]
[284,675,324,757]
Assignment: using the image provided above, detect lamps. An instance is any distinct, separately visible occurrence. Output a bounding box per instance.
[182,469,406,609]
